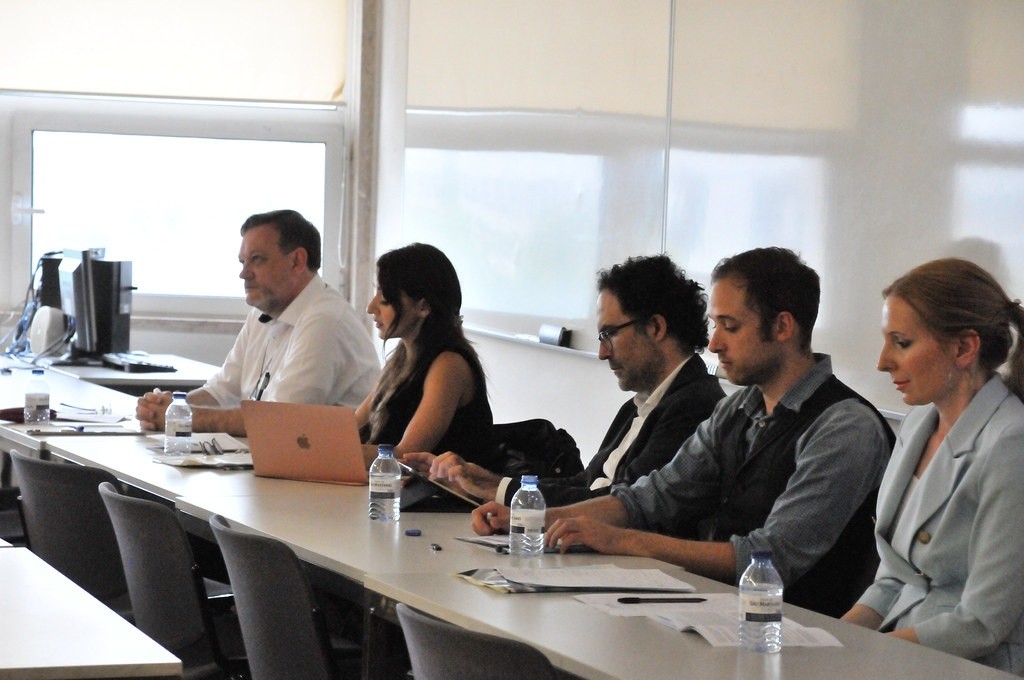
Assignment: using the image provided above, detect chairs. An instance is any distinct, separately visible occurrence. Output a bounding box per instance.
[392,601,559,679]
[96,481,362,680]
[9,450,134,621]
[208,512,409,679]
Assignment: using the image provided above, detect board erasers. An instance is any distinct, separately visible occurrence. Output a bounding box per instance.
[538,325,573,348]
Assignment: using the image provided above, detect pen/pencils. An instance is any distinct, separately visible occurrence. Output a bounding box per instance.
[70,425,84,431]
[256,372,270,401]
[154,387,161,394]
[617,597,709,604]
[487,512,492,524]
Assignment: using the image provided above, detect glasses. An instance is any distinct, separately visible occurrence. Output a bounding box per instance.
[596,318,644,352]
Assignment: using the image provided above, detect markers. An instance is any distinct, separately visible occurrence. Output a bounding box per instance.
[496,544,509,555]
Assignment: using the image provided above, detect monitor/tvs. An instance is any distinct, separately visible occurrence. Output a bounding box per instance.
[48,248,100,366]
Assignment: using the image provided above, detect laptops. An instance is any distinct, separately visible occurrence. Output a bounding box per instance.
[239,399,411,485]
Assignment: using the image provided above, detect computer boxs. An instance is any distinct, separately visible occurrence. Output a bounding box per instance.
[39,254,133,358]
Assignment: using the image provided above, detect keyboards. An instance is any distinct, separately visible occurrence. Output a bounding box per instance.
[102,352,178,374]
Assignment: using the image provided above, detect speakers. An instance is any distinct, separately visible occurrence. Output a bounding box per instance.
[29,305,67,357]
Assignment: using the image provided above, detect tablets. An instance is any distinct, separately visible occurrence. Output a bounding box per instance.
[397,461,486,508]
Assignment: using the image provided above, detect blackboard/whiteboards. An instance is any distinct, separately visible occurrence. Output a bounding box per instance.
[376,0,1024,420]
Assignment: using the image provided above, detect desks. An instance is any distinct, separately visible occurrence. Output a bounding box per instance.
[0,351,1024,680]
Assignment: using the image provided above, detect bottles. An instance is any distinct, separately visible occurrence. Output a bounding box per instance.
[24,370,50,426]
[165,394,193,457]
[739,550,784,654]
[369,444,401,522]
[510,476,546,559]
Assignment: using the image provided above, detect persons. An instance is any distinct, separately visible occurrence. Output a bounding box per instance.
[136,210,381,437]
[840,258,1024,680]
[472,247,897,619]
[404,255,728,508]
[355,242,493,476]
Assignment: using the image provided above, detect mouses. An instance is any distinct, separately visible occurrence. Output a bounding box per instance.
[131,350,149,357]
